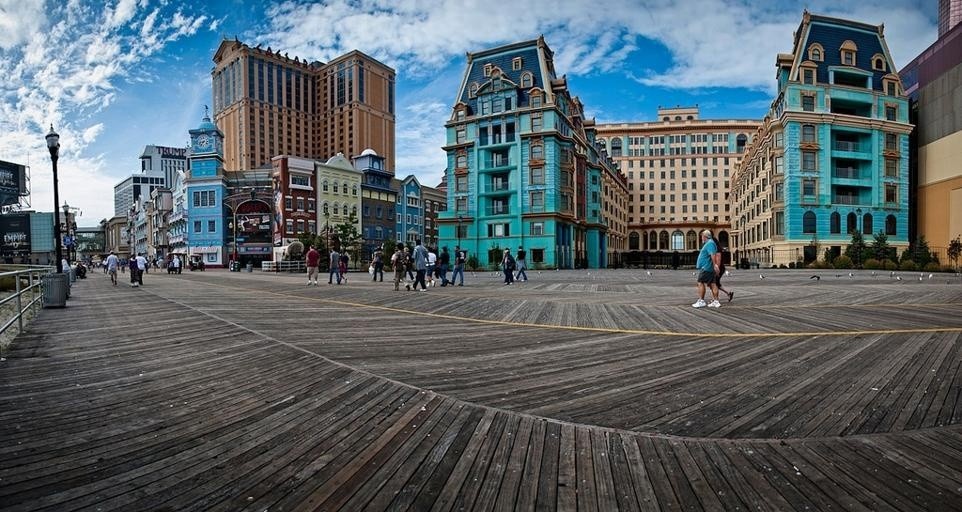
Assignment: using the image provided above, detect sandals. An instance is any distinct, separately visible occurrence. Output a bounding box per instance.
[728,292,733,302]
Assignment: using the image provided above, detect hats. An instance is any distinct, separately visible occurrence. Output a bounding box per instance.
[702,230,712,238]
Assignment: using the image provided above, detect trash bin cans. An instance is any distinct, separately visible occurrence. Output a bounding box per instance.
[70,269,76,282]
[62,270,70,300]
[247,263,253,272]
[229,260,240,272]
[43,274,67,309]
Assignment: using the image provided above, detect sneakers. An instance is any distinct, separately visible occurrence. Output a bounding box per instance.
[707,302,722,308]
[692,299,706,308]
[420,279,436,292]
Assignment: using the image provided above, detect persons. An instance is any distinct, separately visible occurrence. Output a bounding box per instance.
[306,238,467,292]
[708,230,733,303]
[690,229,721,308]
[497,249,516,286]
[128,254,139,286]
[514,245,528,282]
[136,252,149,285]
[504,248,516,284]
[106,250,120,286]
[70,253,183,280]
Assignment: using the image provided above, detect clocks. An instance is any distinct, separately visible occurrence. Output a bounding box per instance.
[196,133,210,150]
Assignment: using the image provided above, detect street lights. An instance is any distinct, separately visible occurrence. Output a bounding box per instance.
[324,210,331,265]
[44,123,66,273]
[61,199,73,265]
[126,239,136,258]
[457,214,463,252]
